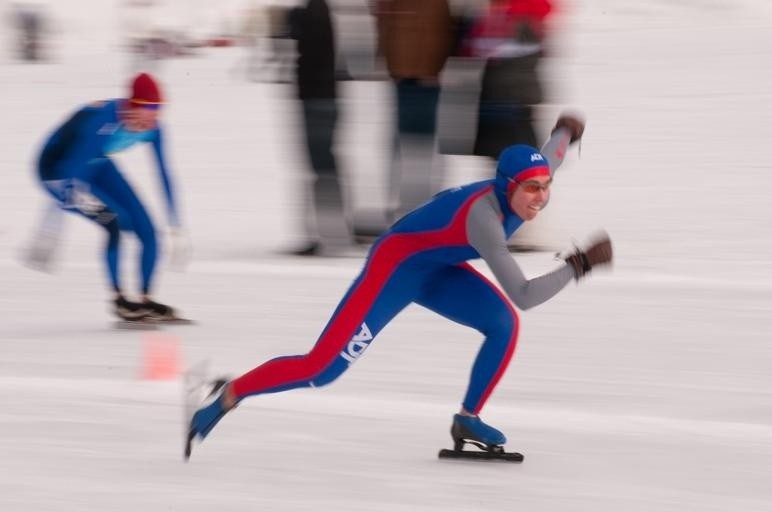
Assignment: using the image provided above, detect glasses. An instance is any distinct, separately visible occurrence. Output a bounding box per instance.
[509,175,556,195]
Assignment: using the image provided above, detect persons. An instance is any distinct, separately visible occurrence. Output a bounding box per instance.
[282,2,345,236]
[475,1,554,155]
[363,2,455,232]
[192,112,614,452]
[36,74,183,321]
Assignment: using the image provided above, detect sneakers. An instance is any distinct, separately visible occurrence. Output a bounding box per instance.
[140,299,175,320]
[191,377,231,444]
[450,411,506,446]
[112,296,154,319]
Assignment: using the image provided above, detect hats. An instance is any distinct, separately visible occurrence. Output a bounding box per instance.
[132,72,165,107]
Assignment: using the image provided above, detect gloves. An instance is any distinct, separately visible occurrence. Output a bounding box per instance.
[565,237,615,281]
[551,108,587,146]
